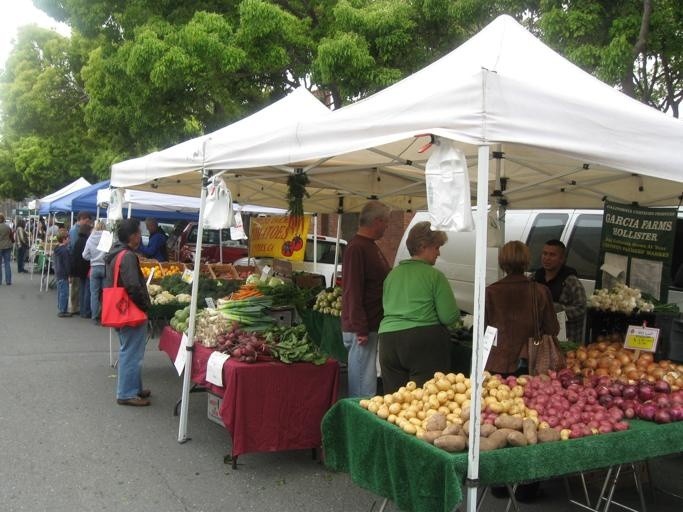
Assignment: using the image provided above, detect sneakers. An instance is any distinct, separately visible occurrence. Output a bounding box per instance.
[116,389,152,407]
[55,310,100,326]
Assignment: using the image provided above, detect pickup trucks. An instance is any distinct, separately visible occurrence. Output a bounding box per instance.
[231,233,349,287]
[139,221,250,267]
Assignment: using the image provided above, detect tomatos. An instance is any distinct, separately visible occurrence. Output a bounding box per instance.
[282,241,293,256]
[292,237,302,251]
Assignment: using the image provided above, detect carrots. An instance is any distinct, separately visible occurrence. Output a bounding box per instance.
[230,284,262,300]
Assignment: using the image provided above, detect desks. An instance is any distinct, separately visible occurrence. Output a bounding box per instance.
[160,324,339,470]
[144,301,169,346]
[305,294,474,376]
[580,299,682,360]
[320,346,683,512]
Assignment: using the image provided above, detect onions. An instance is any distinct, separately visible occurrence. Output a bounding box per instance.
[558,336,683,423]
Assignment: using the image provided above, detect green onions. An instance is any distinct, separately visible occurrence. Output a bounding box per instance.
[195,295,272,348]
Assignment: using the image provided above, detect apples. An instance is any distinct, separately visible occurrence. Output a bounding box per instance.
[359,371,628,451]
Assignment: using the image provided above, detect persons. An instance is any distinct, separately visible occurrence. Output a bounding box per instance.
[376,220,462,398]
[341,201,391,400]
[99,219,152,407]
[485,240,560,379]
[528,239,588,345]
[0,210,169,326]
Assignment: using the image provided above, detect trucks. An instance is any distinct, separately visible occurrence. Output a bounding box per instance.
[388,208,682,364]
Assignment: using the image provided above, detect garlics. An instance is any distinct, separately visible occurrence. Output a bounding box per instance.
[589,283,654,313]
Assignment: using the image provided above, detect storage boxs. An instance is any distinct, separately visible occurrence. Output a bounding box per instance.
[201,258,292,281]
[137,257,193,307]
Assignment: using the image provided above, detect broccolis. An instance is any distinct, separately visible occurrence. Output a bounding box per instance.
[147,284,192,305]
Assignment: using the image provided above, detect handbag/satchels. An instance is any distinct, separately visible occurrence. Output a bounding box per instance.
[527,333,567,376]
[100,287,148,329]
